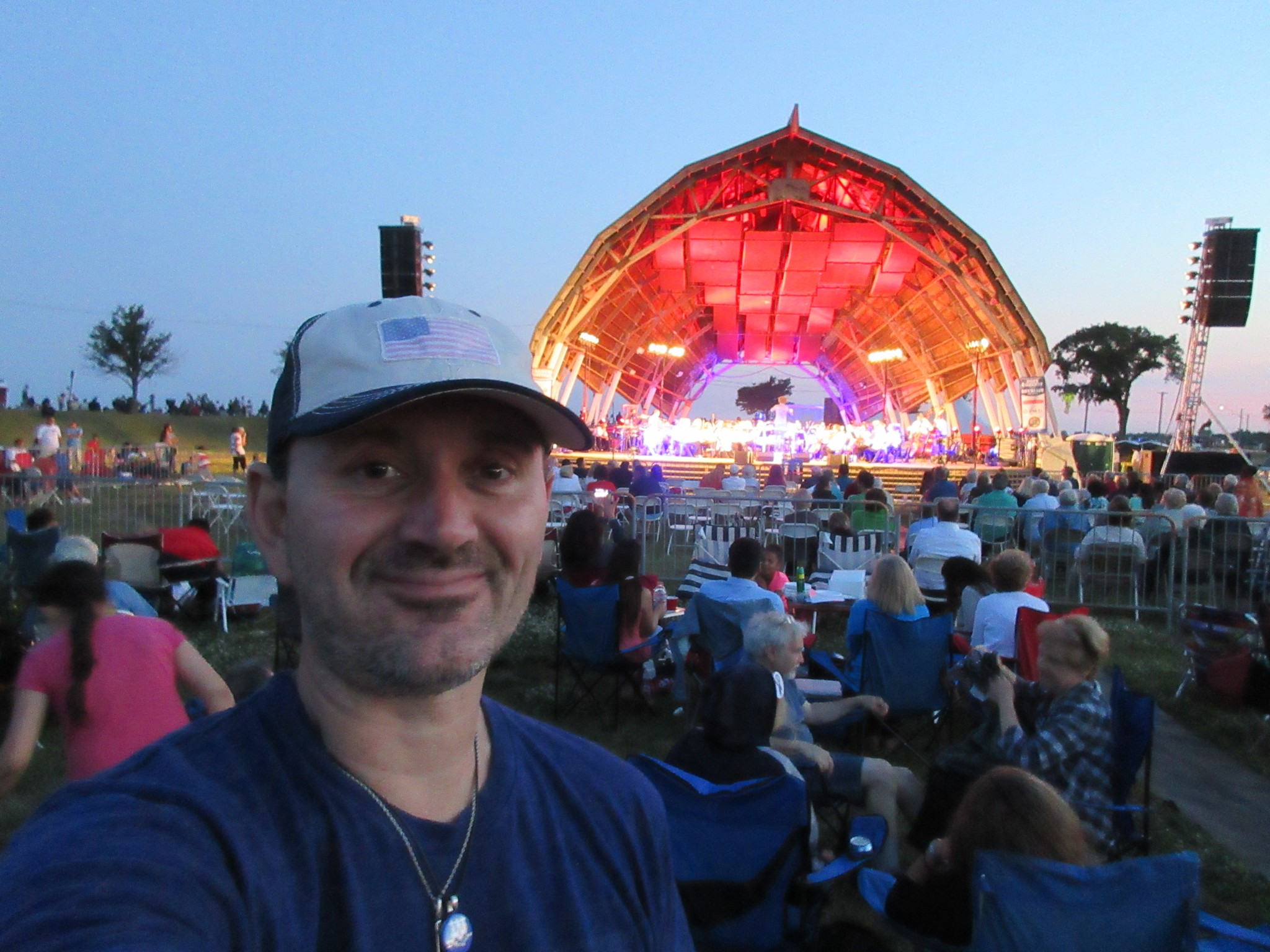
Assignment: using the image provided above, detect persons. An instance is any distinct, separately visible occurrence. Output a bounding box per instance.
[753,543,795,616]
[905,614,1113,873]
[846,554,932,757]
[737,611,924,882]
[696,536,786,677]
[942,556,996,646]
[1,561,235,802]
[561,492,622,588]
[969,548,1051,659]
[664,662,831,928]
[591,538,670,700]
[907,498,982,594]
[880,768,1103,951]
[2,379,281,642]
[542,396,1240,577]
[0,296,694,952]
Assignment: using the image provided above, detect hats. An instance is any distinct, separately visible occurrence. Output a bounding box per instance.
[742,466,756,477]
[270,291,595,454]
[810,466,821,471]
[822,469,834,478]
[730,464,740,473]
[932,466,950,480]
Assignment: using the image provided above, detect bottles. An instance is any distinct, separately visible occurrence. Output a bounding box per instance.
[653,582,667,610]
[796,567,805,592]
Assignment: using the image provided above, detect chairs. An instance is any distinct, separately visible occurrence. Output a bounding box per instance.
[546,480,1270,582]
[159,552,231,633]
[543,575,1199,952]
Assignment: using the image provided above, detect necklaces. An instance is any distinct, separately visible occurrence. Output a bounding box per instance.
[316,729,480,952]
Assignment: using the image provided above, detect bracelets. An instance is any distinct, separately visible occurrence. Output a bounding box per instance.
[928,838,941,862]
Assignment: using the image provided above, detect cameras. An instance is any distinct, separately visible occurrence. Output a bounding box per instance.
[967,650,1000,677]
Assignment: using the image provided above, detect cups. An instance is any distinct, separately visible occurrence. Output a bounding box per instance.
[666,596,679,610]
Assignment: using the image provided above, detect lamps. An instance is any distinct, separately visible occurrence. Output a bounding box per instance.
[421,241,436,298]
[1177,241,1201,324]
